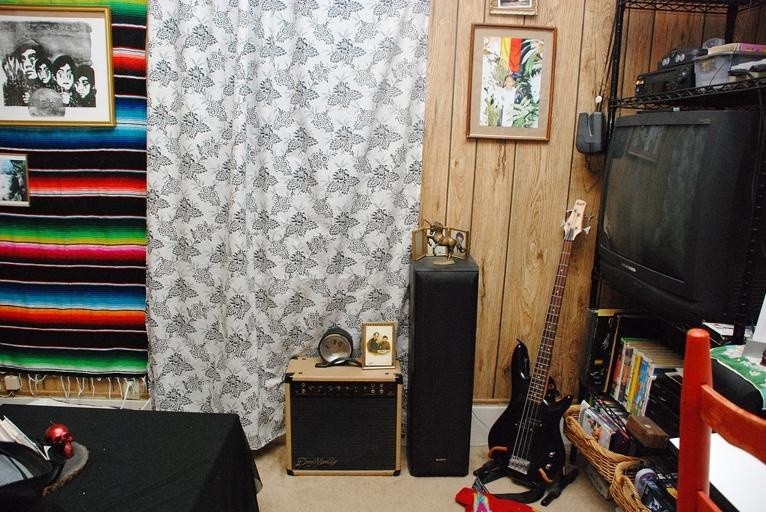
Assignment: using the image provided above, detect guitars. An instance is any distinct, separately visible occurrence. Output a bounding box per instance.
[488,198,592,484]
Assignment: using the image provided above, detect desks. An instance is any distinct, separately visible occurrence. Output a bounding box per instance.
[1,404,262,512]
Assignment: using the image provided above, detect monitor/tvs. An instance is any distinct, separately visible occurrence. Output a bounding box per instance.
[596,108,766,326]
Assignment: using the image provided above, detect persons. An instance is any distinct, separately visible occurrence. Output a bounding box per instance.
[378,336,390,350]
[2,42,97,108]
[14,172,26,201]
[455,232,464,244]
[495,74,524,127]
[367,332,380,353]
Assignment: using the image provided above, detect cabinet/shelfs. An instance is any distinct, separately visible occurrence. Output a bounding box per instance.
[567,0,766,465]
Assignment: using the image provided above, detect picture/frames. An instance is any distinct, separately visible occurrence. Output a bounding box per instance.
[466,23,557,141]
[0,153,31,208]
[361,321,399,369]
[1,4,116,128]
[411,226,469,261]
[487,1,539,16]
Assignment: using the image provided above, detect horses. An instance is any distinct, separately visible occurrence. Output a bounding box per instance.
[426,221,462,261]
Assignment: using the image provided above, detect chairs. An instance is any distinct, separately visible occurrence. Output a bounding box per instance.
[675,327,766,512]
[669,432,765,512]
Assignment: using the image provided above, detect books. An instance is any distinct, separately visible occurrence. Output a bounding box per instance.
[707,42,766,54]
[579,337,685,458]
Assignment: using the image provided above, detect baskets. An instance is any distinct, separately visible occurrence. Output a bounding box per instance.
[608,460,655,512]
[563,404,646,483]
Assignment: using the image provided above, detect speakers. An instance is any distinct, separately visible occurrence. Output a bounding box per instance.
[406,250,479,477]
